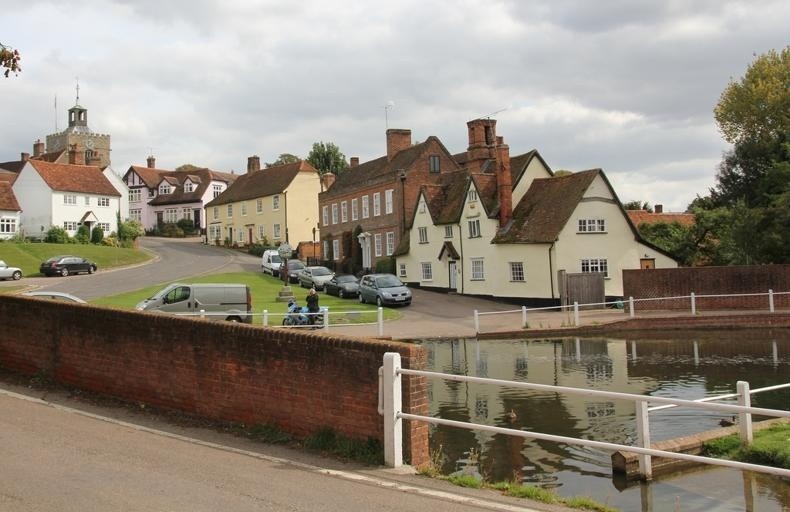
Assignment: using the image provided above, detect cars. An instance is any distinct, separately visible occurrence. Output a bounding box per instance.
[324,272,360,299]
[299,266,335,291]
[40,255,98,277]
[0,258,22,280]
[22,291,87,304]
[278,259,306,283]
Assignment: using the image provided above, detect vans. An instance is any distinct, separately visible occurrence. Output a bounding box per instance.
[137,282,252,326]
[261,249,284,276]
[357,273,413,308]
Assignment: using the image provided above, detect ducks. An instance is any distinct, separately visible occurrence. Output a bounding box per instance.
[720,416,737,426]
[507,408,517,420]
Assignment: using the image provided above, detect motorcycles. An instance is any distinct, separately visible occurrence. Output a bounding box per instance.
[282,298,327,330]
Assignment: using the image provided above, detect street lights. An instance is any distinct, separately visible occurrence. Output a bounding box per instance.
[278,240,292,286]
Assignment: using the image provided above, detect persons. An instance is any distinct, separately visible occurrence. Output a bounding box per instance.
[288,299,303,321]
[306,288,320,329]
